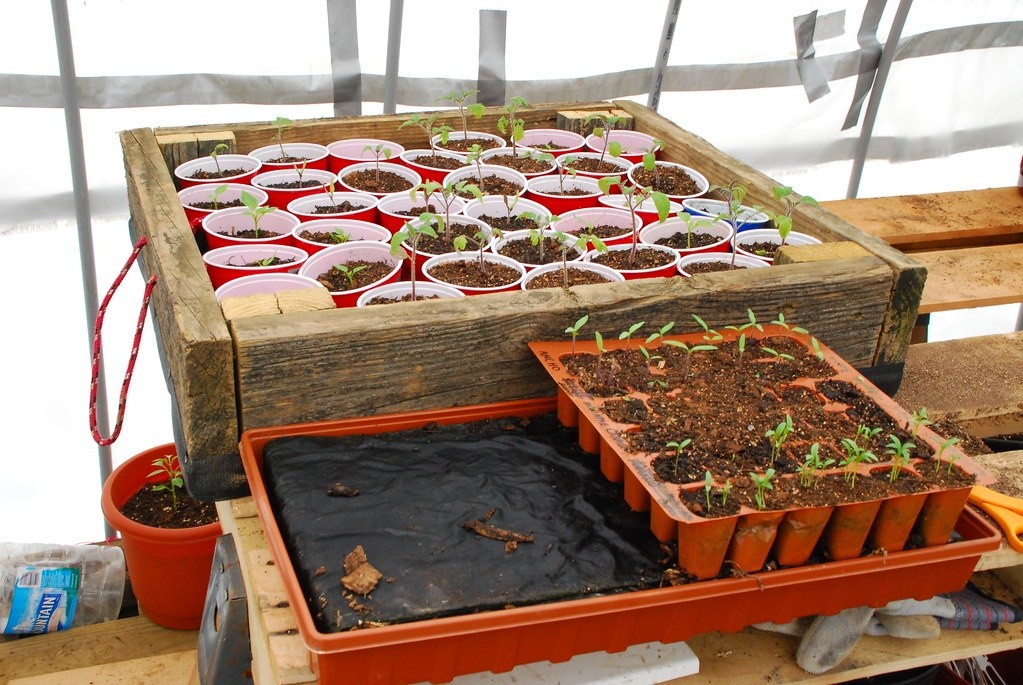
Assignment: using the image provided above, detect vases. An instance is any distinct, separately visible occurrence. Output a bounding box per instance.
[235,399,1001,685]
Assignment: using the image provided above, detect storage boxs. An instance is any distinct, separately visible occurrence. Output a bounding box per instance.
[117,100,928,503]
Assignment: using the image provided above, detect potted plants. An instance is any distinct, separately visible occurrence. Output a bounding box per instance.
[102,442,226,634]
[172,96,993,580]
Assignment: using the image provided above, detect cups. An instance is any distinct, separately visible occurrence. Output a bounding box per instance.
[172,128,823,322]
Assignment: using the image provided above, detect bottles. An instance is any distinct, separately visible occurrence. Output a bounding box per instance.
[0,539,126,637]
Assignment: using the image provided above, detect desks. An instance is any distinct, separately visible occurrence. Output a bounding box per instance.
[215,185,1023,685]
